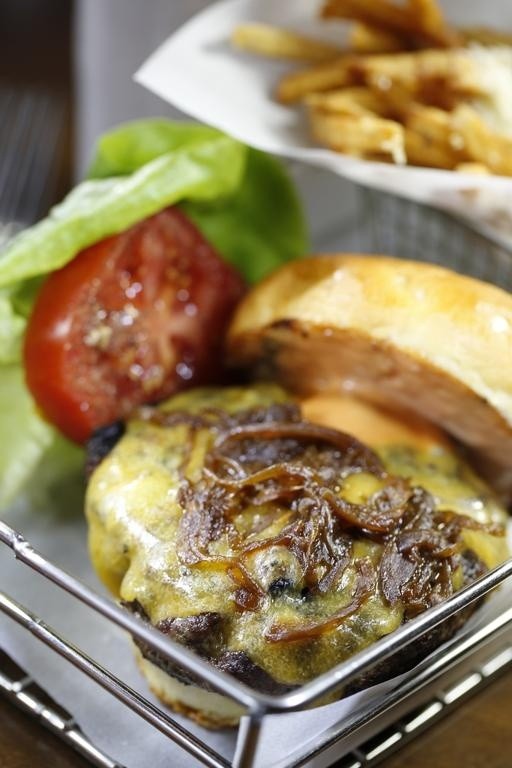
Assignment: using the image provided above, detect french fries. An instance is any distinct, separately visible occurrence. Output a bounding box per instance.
[231,0,511,195]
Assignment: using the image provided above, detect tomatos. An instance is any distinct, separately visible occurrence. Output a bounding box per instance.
[23,204,251,450]
